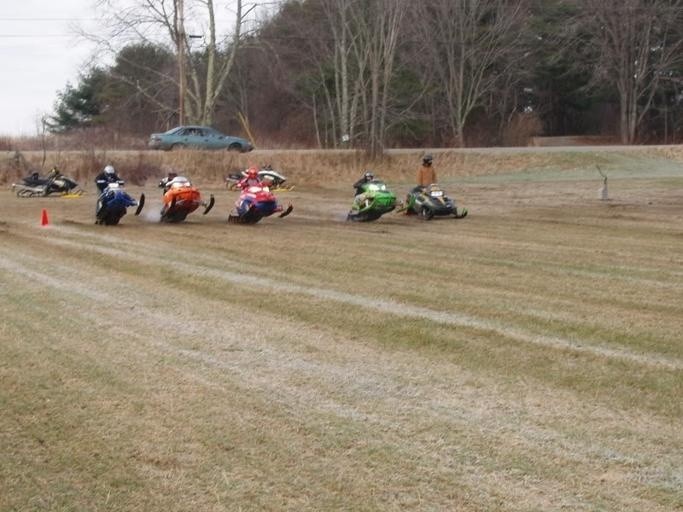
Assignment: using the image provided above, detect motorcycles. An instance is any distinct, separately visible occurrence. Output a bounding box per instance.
[94,180,146,225]
[11,167,78,197]
[226,170,293,224]
[347,179,468,222]
[160,176,216,223]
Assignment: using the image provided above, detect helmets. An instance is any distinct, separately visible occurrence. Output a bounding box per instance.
[104,165,116,174]
[168,172,177,181]
[423,155,432,165]
[364,171,373,181]
[249,167,258,179]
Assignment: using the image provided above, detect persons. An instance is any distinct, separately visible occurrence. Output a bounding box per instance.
[237,167,260,189]
[417,154,438,185]
[95,165,121,191]
[158,172,177,194]
[353,172,374,195]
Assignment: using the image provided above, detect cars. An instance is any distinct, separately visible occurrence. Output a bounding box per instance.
[147,124,254,154]
